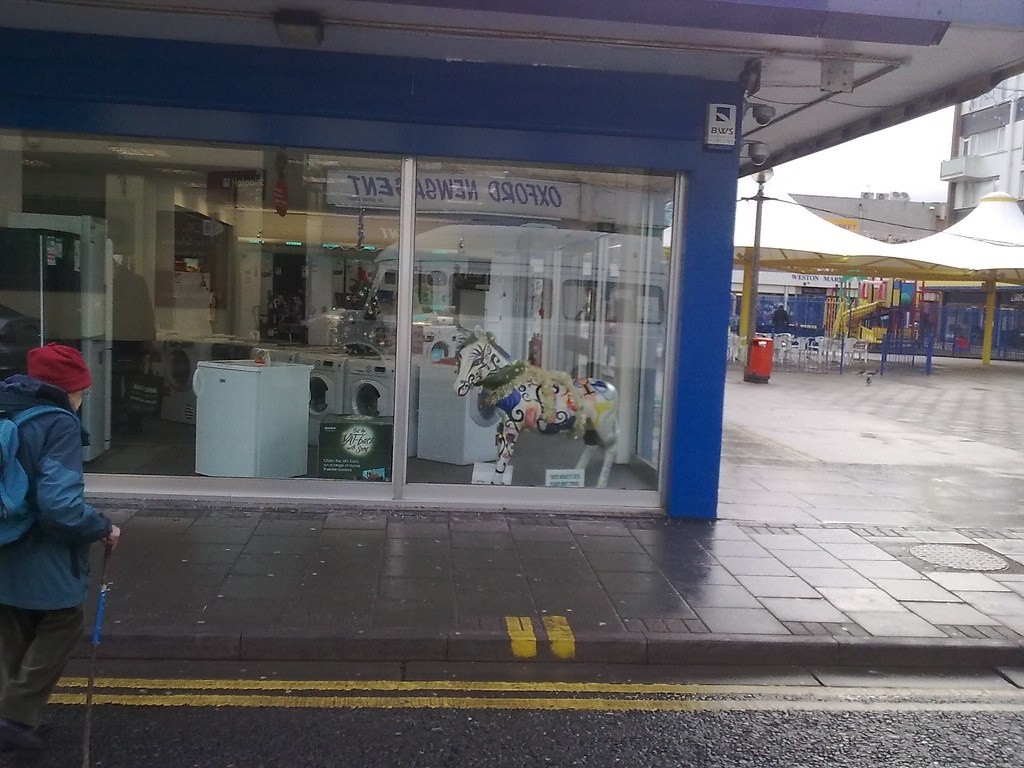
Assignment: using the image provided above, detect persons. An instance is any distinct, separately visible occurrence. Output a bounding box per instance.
[1,344,122,761]
[770,303,789,339]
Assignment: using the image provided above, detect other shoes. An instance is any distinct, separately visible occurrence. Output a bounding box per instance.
[0,722,51,754]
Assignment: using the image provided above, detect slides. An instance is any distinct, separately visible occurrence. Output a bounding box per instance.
[841,298,881,346]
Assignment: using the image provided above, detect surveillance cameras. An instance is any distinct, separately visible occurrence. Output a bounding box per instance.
[748,142,769,167]
[753,105,776,127]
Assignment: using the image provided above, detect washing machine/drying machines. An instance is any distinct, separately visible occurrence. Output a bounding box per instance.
[140,318,530,473]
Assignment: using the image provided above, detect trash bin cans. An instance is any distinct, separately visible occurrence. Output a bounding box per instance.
[746,337,774,385]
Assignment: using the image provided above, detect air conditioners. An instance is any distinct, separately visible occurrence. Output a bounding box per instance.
[876,193,890,199]
[860,192,875,199]
[889,191,911,200]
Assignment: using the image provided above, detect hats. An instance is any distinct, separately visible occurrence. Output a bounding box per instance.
[26,342,93,396]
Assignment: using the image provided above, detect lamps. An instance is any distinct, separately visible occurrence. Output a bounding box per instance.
[272,6,325,46]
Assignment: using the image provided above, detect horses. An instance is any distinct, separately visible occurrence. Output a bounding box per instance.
[451,324,620,489]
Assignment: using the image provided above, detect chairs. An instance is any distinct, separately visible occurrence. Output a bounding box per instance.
[728,328,871,371]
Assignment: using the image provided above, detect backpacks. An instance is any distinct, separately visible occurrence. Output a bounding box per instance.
[0,406,83,546]
